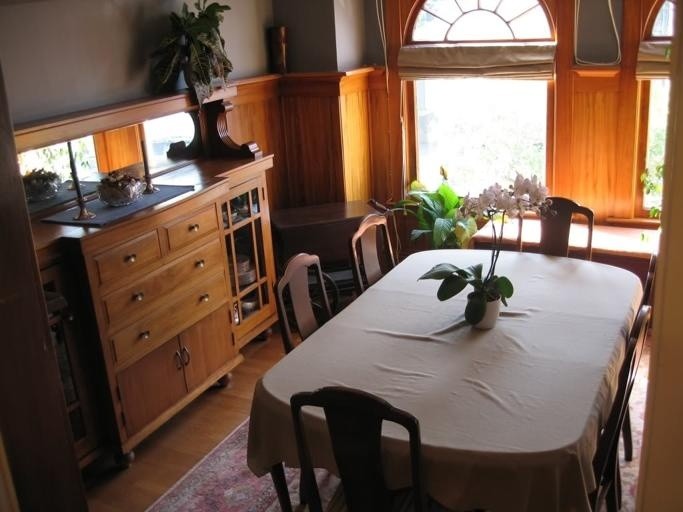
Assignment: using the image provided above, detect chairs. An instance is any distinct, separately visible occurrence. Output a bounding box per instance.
[276,196,657,511]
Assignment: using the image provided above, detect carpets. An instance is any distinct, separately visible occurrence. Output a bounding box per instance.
[139,363,649,511]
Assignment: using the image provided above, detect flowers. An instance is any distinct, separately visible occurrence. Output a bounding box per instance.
[416,173,557,325]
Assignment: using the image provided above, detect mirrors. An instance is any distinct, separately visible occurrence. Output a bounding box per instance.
[14,111,200,216]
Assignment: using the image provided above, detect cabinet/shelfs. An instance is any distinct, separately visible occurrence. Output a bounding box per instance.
[30,152,274,470]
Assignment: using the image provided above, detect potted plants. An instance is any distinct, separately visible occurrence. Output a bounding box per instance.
[145,0,234,117]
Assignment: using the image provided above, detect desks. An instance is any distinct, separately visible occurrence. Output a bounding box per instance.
[466,213,658,328]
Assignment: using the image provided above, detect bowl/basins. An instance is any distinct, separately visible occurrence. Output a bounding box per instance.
[24,176,62,201]
[94,181,147,207]
